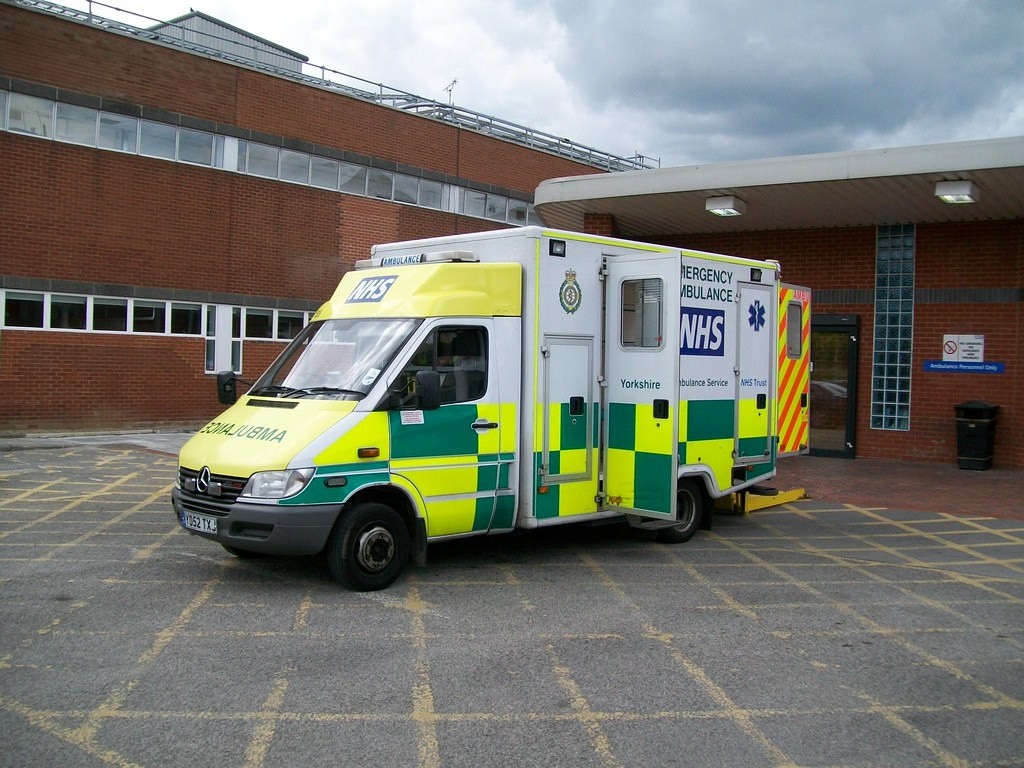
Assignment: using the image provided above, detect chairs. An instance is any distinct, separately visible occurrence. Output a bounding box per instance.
[441,331,484,400]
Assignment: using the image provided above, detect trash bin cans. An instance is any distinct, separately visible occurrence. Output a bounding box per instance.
[953,399,1000,471]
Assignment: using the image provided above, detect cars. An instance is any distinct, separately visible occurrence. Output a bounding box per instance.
[811,381,847,428]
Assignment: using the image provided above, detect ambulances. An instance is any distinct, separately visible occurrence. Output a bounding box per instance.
[170,224,814,591]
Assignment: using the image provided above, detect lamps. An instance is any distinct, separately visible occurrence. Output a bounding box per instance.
[705,195,747,217]
[935,181,980,204]
